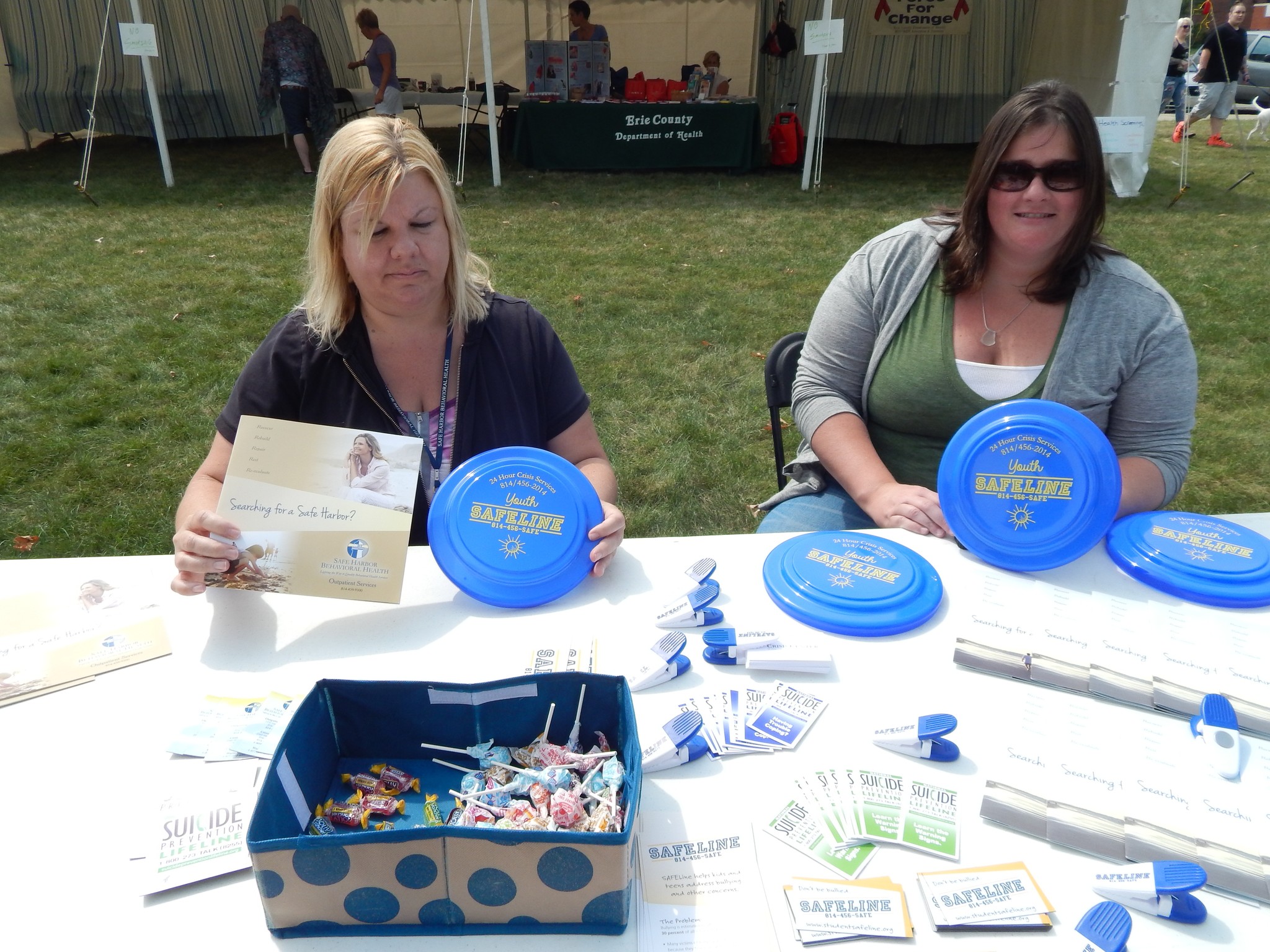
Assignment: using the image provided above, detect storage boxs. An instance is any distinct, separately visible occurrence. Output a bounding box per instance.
[244,668,646,935]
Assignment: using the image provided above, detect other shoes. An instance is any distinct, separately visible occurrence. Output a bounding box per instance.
[1184,131,1196,138]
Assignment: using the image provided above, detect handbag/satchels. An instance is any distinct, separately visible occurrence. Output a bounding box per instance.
[763,1,797,59]
[626,71,688,102]
[332,88,354,103]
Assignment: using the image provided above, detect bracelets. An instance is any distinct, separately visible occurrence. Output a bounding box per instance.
[359,61,361,66]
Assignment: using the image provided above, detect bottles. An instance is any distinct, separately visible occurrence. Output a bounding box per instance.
[469,73,475,91]
[527,92,559,100]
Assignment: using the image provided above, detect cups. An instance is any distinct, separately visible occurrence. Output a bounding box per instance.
[418,81,429,92]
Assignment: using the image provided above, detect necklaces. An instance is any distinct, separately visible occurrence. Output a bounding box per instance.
[980,282,1036,346]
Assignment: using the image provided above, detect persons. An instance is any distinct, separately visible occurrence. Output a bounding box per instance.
[568,0,611,61]
[1173,3,1250,148]
[171,116,625,595]
[257,5,338,175]
[702,50,729,100]
[347,8,404,119]
[1159,17,1196,138]
[747,78,1197,539]
[346,433,418,500]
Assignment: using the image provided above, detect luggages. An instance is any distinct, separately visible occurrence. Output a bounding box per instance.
[768,103,804,172]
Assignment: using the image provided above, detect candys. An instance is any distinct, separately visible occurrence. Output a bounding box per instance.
[307,683,630,838]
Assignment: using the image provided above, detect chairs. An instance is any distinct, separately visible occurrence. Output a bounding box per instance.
[458,87,508,157]
[330,88,375,127]
[396,78,424,129]
[762,331,813,490]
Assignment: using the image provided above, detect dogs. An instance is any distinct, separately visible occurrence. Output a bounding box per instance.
[1246,95,1270,142]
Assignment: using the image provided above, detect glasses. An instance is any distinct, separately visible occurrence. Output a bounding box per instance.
[364,51,369,59]
[1182,25,1193,29]
[989,161,1087,192]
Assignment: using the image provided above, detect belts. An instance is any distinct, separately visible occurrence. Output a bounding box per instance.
[281,85,310,91]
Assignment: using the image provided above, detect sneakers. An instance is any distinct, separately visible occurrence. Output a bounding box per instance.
[1173,121,1187,144]
[1208,132,1233,148]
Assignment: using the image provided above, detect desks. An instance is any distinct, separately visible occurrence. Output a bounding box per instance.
[498,94,762,174]
[0,507,1270,949]
[334,89,527,121]
